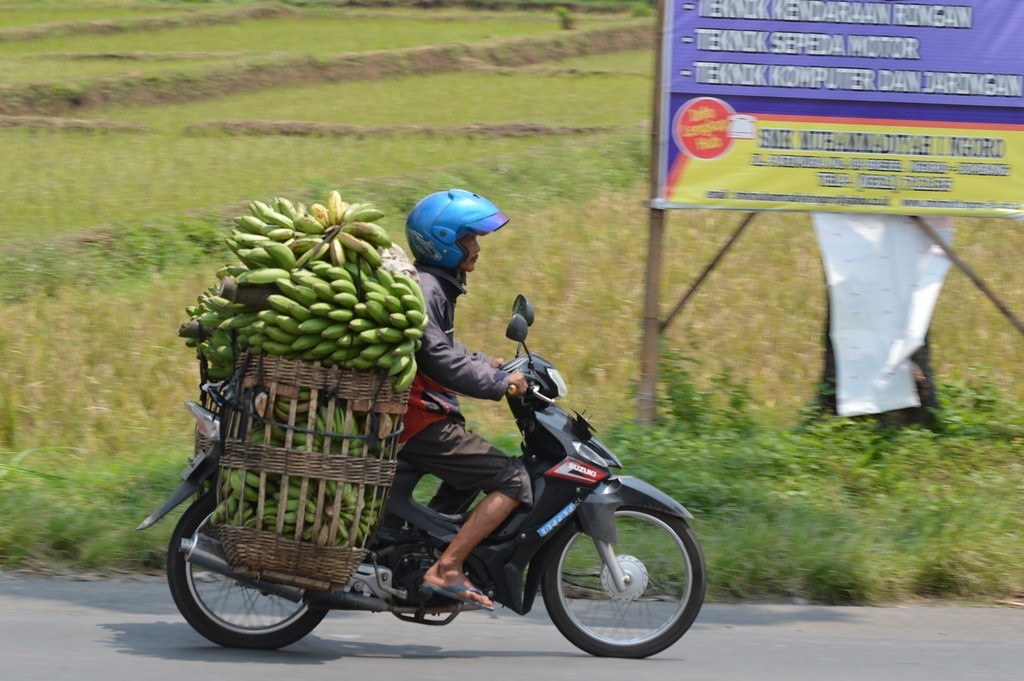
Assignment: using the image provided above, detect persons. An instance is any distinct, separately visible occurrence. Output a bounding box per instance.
[394,188,534,612]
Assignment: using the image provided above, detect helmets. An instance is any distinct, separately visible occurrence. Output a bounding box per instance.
[405,189,510,275]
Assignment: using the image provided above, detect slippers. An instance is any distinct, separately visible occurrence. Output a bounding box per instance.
[423,582,494,611]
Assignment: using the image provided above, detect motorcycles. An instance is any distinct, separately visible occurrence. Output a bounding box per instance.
[135,294,708,658]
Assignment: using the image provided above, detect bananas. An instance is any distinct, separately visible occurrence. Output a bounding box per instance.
[183,191,434,549]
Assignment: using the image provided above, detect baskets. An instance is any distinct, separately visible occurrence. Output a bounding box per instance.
[193,352,411,591]
